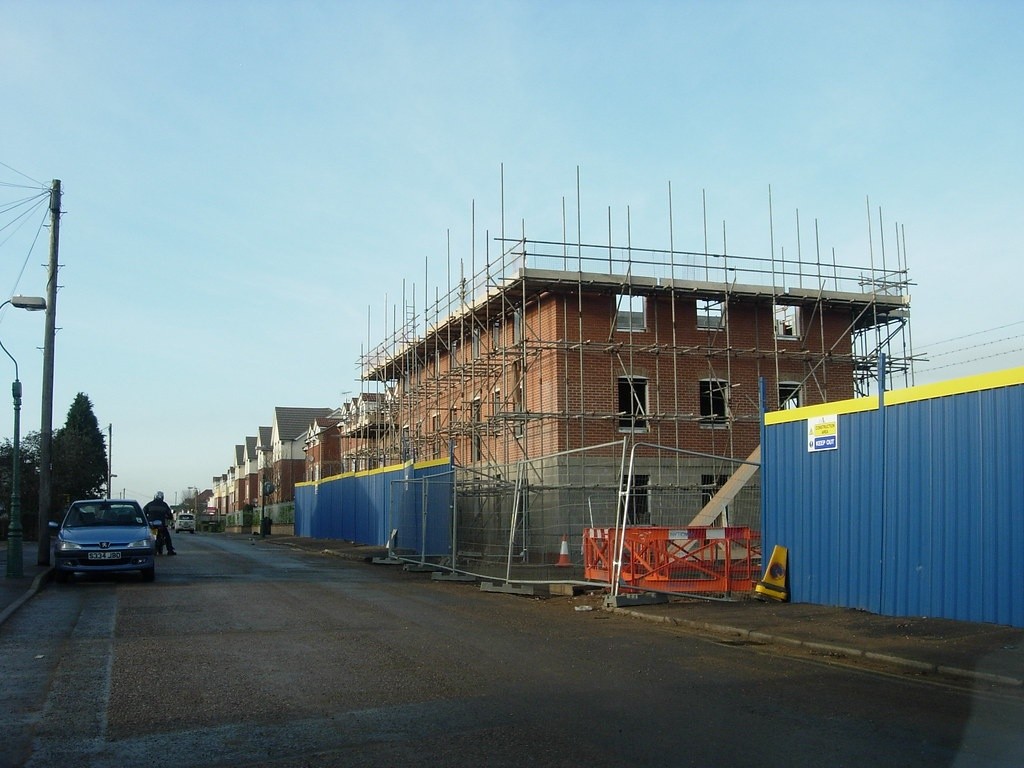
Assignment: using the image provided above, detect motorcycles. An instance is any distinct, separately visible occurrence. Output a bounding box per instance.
[148,517,168,555]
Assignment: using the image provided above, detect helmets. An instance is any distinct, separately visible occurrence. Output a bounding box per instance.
[154,491,164,501]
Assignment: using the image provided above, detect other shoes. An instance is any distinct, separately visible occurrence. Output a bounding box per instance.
[167,551,176,555]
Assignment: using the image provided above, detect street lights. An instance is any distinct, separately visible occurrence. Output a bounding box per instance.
[0,293,47,579]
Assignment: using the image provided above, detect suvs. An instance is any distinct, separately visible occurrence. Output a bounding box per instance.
[175,513,196,534]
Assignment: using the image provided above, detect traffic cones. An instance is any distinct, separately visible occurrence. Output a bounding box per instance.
[553,534,573,566]
[754,544,788,601]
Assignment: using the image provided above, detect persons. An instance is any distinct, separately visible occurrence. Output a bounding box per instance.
[143,491,178,556]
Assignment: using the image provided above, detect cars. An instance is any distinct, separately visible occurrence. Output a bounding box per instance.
[48,499,163,582]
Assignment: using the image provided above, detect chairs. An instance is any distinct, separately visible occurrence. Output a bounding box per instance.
[117,514,134,523]
[77,512,95,523]
